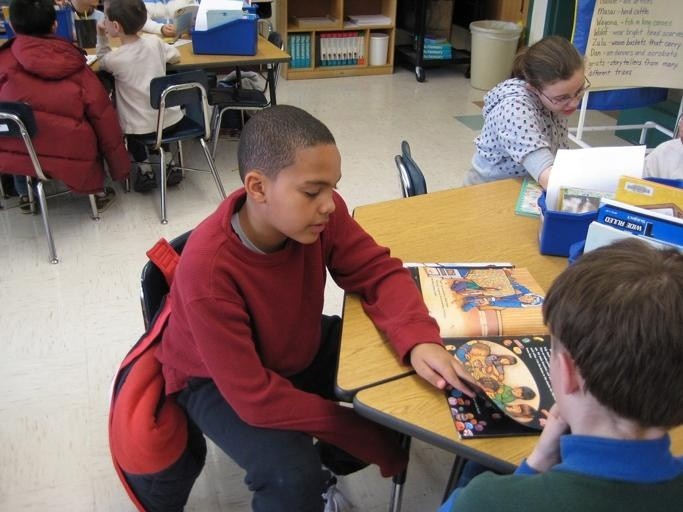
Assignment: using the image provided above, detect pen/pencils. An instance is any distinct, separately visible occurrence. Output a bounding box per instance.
[84,10,88,20]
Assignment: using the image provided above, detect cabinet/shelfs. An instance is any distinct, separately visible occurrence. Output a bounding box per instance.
[273,0,397,80]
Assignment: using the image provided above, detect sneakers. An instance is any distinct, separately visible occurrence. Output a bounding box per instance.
[19,196,38,213]
[96,187,115,213]
[135,167,156,191]
[166,165,182,186]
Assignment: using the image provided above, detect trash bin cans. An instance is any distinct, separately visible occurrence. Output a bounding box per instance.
[469,20,521,91]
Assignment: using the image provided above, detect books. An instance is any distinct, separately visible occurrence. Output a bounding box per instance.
[403,261,556,440]
[316,31,366,65]
[288,32,310,68]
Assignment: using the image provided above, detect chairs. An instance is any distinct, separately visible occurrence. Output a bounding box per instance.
[124,70,229,224]
[394,141,427,197]
[0,101,100,264]
[140,228,194,332]
[208,32,285,162]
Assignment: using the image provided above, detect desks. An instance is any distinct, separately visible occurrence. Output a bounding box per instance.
[73,32,292,105]
[335,173,683,512]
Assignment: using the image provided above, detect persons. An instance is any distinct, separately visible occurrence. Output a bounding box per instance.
[437,236,682,511]
[96,0,186,194]
[460,34,586,193]
[68,1,105,41]
[642,113,683,179]
[152,104,481,511]
[139,0,199,38]
[1,0,133,215]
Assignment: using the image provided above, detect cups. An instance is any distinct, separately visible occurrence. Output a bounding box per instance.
[368,32,389,66]
[73,18,97,48]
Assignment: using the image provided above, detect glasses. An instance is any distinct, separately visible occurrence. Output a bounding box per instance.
[537,76,591,104]
